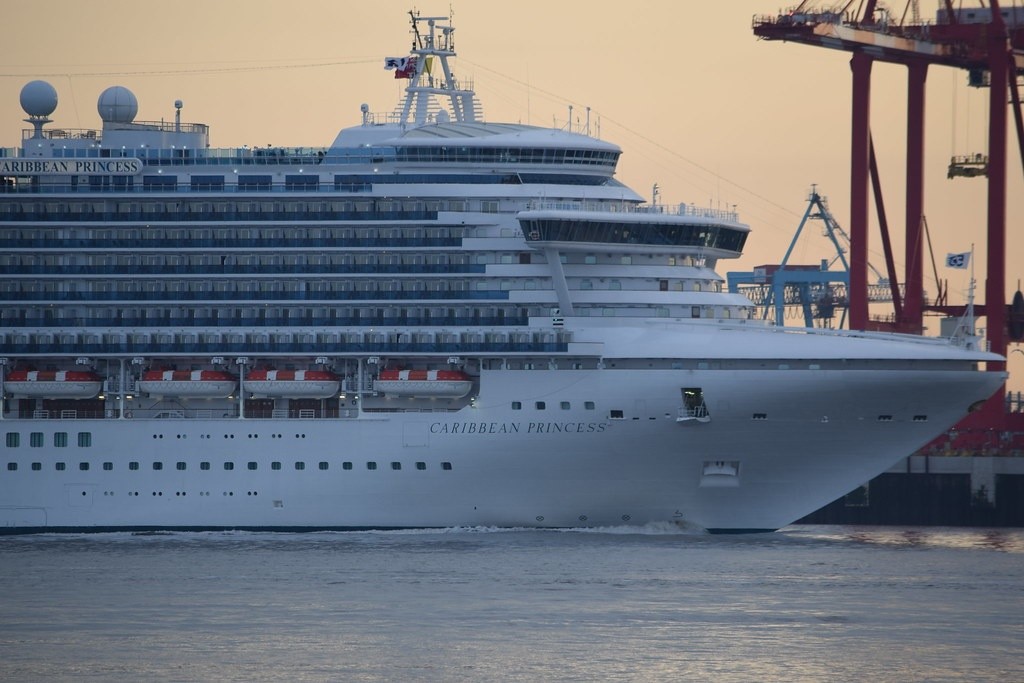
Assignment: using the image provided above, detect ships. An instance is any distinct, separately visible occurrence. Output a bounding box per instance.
[0,11,1008,536]
